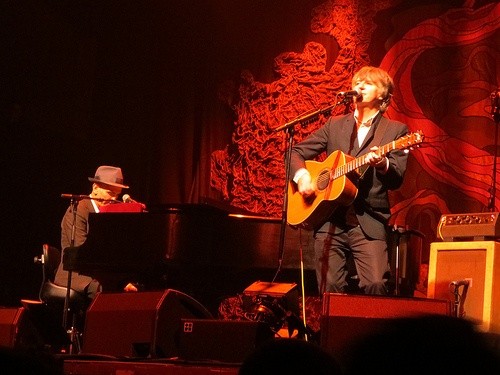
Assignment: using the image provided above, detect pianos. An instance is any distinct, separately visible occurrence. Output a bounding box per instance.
[63,204,317,294]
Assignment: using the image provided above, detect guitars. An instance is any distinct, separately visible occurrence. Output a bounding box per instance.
[286,131,417,231]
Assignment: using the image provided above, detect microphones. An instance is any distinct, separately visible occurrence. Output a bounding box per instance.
[122,194,147,209]
[450,280,469,286]
[338,88,362,98]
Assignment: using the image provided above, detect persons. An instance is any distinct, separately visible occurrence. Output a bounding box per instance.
[53,166,130,303]
[236,314,500,375]
[283,67,409,298]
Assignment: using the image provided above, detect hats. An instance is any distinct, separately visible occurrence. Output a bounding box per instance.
[88,165,129,188]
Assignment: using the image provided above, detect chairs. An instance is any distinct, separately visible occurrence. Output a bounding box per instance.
[39,243,83,353]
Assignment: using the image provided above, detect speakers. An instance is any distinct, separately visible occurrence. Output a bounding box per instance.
[0,307,40,349]
[427,242,500,335]
[81,289,215,361]
[175,318,272,363]
[318,291,452,361]
